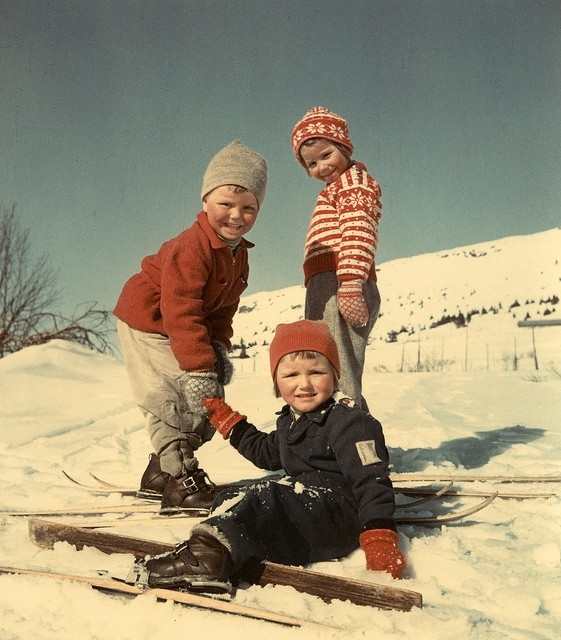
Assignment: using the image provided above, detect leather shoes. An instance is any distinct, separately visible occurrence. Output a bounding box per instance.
[135,529,236,594]
[159,468,221,516]
[137,452,171,500]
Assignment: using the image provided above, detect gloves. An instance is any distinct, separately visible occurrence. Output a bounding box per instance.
[202,397,248,440]
[185,372,221,417]
[337,278,370,328]
[210,340,234,385]
[360,528,407,579]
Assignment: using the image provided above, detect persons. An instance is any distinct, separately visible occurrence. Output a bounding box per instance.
[291,106,381,413]
[136,319,405,602]
[112,138,267,520]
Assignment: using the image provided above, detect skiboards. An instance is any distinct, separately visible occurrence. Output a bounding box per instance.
[59,469,263,498]
[3,477,498,528]
[389,471,561,499]
[0,517,424,630]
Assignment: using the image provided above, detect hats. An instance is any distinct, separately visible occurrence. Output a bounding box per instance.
[292,105,354,168]
[269,319,341,382]
[201,139,268,211]
